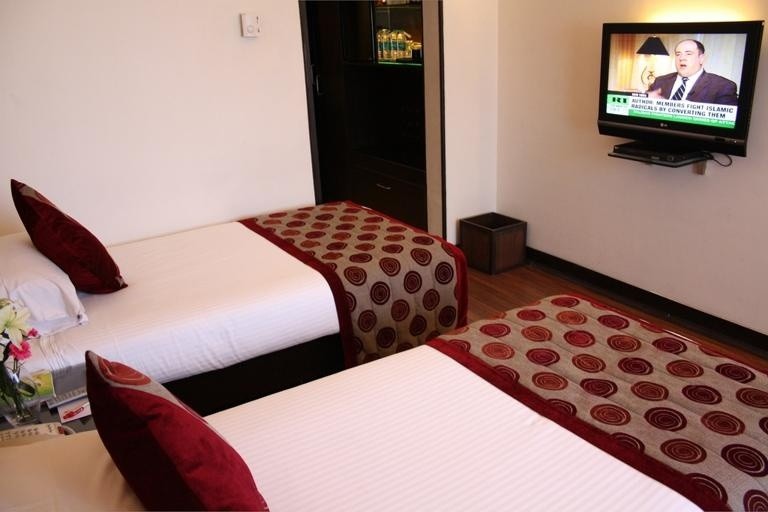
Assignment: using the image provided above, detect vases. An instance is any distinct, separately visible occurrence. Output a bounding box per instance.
[0,354,41,428]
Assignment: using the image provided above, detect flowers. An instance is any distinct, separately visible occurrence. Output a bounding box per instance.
[0,297,41,409]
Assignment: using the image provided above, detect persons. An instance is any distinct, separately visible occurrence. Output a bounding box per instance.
[646,39,737,106]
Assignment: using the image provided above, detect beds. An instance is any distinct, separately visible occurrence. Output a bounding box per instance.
[0,290,768,512]
[0,199,468,417]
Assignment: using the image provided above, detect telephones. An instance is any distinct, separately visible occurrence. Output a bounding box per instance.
[0,422,65,442]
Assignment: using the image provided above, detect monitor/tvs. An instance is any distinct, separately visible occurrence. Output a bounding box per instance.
[597,20,764,167]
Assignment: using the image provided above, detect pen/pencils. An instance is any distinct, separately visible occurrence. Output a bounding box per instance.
[63,401,90,419]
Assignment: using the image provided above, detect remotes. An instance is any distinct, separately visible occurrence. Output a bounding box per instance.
[46,386,87,409]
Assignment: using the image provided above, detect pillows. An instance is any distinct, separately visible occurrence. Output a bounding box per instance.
[11,179,128,294]
[85,351,269,512]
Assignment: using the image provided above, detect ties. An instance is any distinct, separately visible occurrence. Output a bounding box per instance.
[671,77,688,100]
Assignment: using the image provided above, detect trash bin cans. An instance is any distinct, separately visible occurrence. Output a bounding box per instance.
[459,211,528,276]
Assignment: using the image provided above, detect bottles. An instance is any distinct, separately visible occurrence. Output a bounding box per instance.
[378,29,412,60]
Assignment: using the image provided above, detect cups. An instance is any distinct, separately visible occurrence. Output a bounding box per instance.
[410,42,422,60]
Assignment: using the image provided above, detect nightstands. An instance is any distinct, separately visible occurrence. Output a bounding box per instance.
[0,362,98,433]
